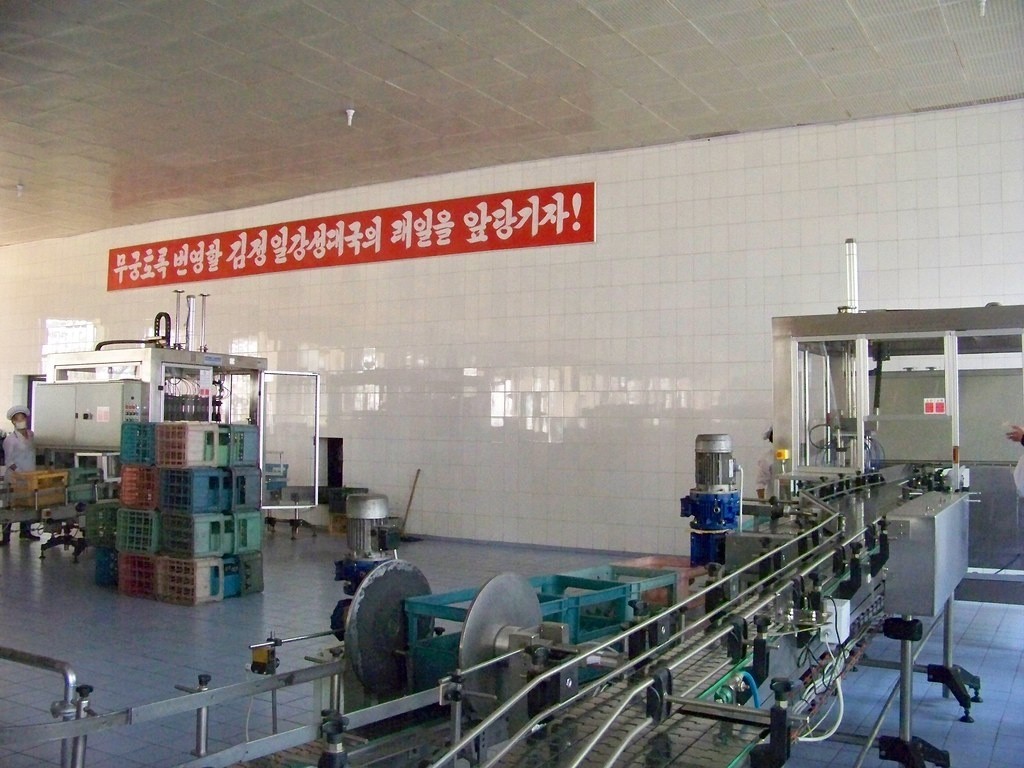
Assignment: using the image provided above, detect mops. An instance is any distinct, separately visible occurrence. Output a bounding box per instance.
[399,469,423,543]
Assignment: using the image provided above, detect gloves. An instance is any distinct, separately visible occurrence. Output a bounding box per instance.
[15,467,26,480]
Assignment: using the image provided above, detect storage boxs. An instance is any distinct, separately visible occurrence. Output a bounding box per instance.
[13,420,264,605]
[404,554,708,720]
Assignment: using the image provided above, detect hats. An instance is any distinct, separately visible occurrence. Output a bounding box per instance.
[7,405,30,420]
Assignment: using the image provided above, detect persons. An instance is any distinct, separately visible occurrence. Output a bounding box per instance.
[1006,425,1024,497]
[0,405,40,545]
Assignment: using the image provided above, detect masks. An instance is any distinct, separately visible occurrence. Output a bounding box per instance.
[13,418,27,430]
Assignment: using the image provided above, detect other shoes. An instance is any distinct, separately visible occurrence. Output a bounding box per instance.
[19,532,40,541]
[0,541,9,545]
